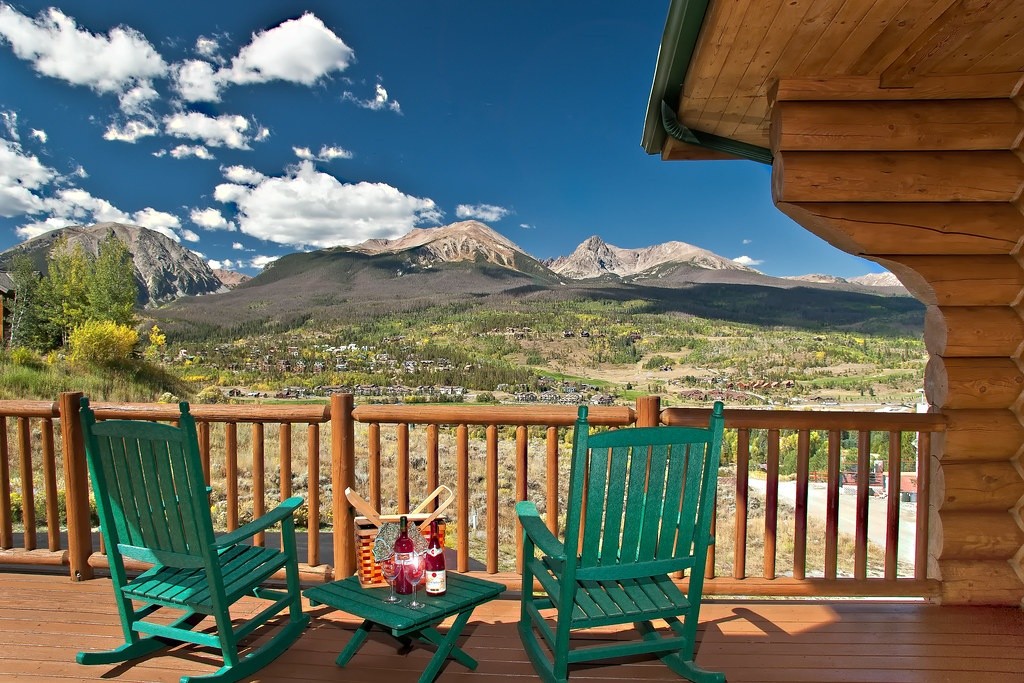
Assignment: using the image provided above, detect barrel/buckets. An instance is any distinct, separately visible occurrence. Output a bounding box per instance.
[902,491,918,502]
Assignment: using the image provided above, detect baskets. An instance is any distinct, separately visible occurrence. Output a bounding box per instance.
[345,485,455,588]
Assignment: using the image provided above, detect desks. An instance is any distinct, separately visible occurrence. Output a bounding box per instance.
[301,570,508,682]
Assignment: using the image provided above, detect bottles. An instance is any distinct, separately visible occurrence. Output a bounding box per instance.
[425,520,446,596]
[394,516,414,595]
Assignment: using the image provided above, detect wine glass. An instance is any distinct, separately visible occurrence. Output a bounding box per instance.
[380,555,401,603]
[402,556,425,609]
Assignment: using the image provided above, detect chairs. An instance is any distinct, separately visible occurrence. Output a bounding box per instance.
[75,396,311,683]
[514,401,728,683]
